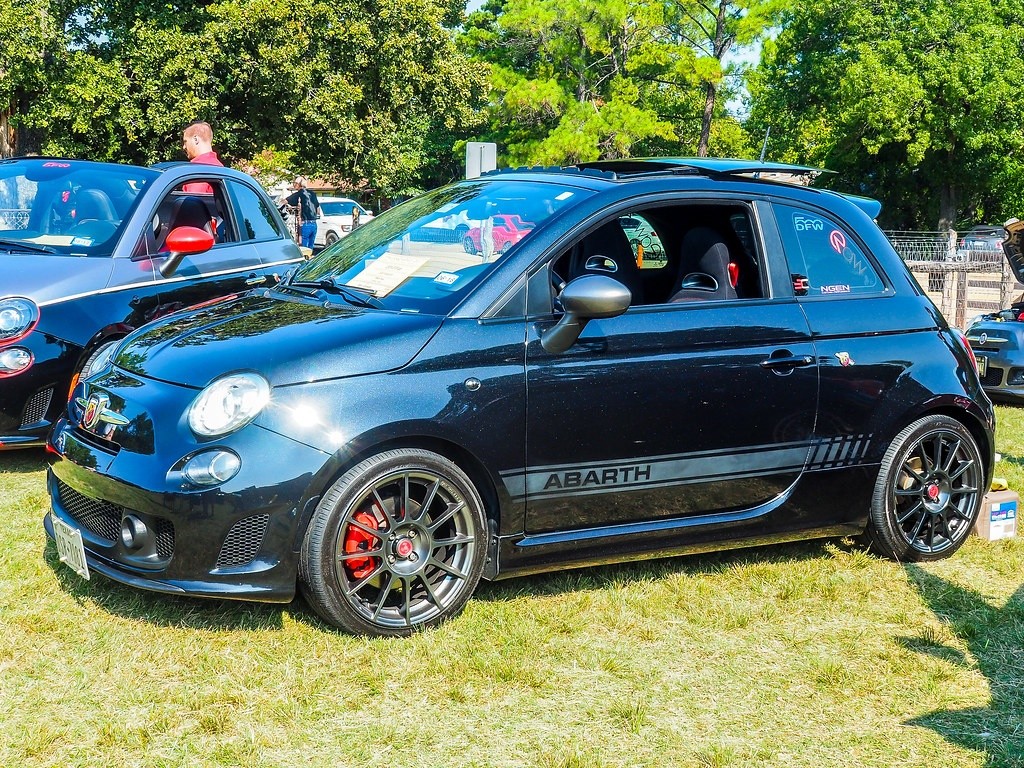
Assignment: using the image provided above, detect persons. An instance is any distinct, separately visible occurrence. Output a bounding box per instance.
[928,218,957,292]
[279,177,320,251]
[182,122,224,193]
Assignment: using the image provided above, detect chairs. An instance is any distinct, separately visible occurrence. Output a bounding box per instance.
[648,227,740,308]
[570,220,641,306]
[154,198,217,255]
[40,188,116,241]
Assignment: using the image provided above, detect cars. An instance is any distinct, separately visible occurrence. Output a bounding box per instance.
[421,209,486,231]
[959,224,1009,255]
[618,215,665,254]
[313,196,376,249]
[462,213,537,255]
[963,217,1024,406]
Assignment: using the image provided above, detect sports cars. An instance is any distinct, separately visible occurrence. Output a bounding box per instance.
[0,154,310,451]
[39,153,998,638]
